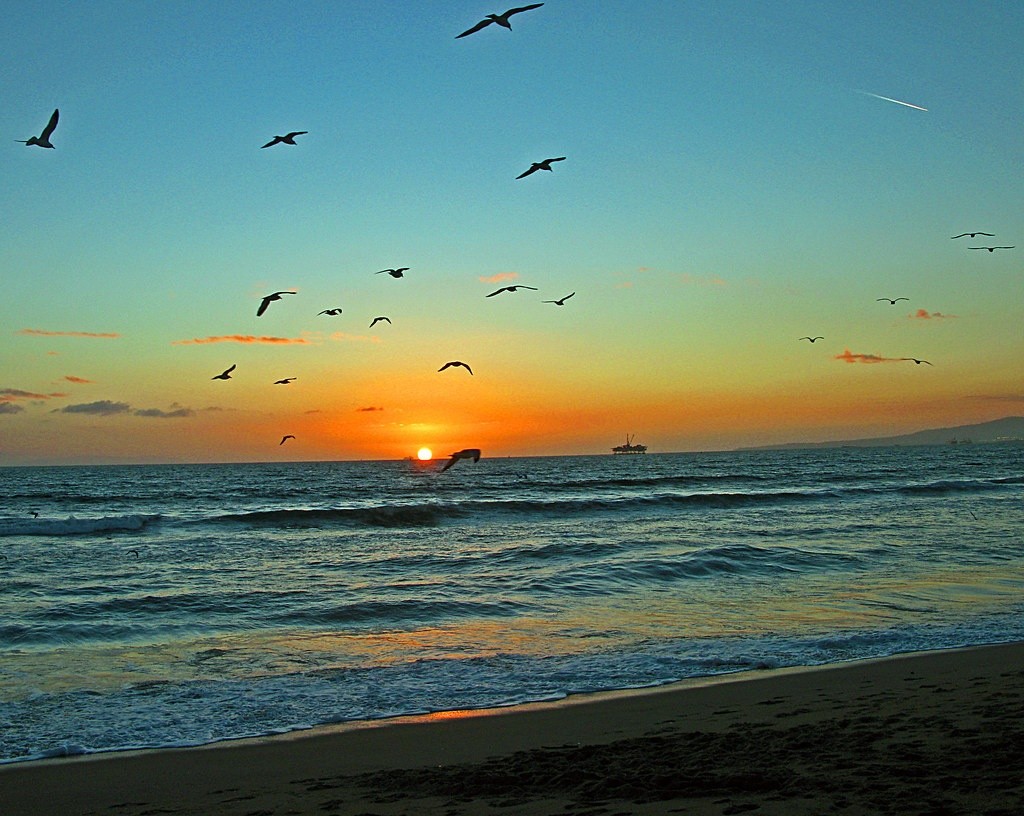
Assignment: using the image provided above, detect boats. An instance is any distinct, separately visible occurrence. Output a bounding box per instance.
[613,433,647,454]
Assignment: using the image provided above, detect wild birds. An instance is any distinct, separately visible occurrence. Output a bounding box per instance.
[454,2,545,40]
[950,232,1016,253]
[260,131,308,149]
[374,268,411,278]
[256,291,391,328]
[485,286,576,306]
[798,337,824,343]
[900,358,934,367]
[437,361,474,375]
[279,435,296,446]
[515,157,566,180]
[211,364,297,385]
[440,449,481,473]
[876,297,909,305]
[13,108,60,150]
